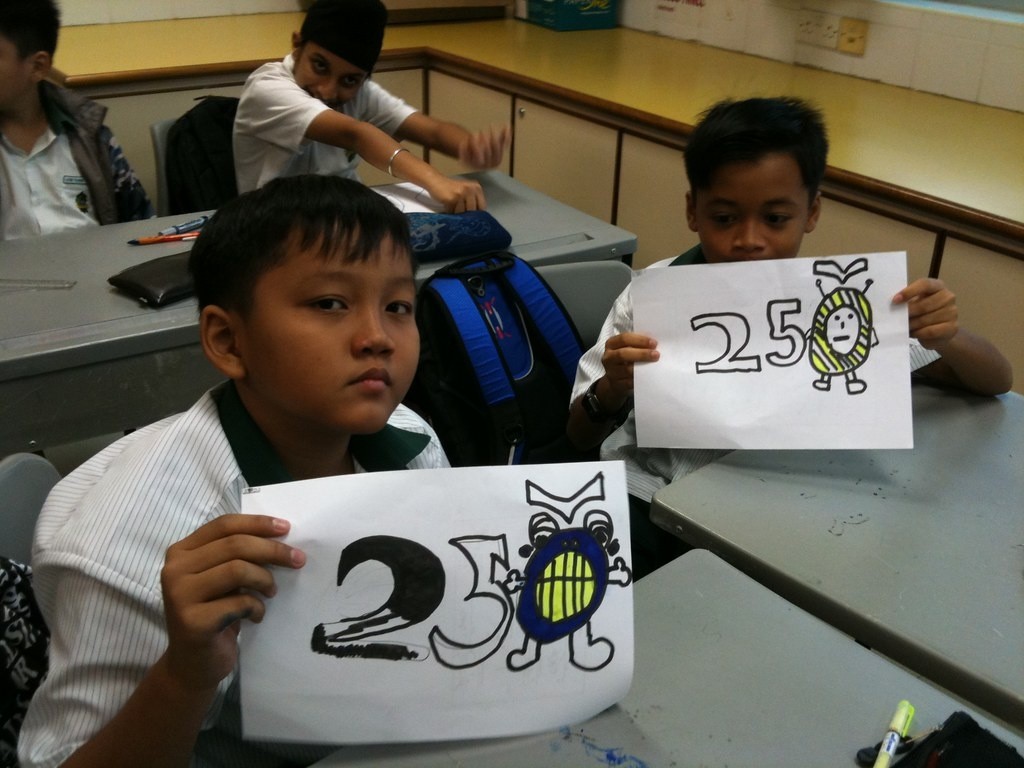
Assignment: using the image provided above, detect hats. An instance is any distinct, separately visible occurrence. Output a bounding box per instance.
[301,0,387,78]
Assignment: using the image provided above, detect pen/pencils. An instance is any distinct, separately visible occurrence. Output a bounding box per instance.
[872,699,915,768]
[126,231,202,244]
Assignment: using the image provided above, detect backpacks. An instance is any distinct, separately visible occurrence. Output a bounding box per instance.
[416,249,588,466]
[165,95,240,215]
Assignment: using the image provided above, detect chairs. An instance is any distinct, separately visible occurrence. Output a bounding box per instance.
[148,94,241,217]
[0,453,61,568]
[531,260,631,345]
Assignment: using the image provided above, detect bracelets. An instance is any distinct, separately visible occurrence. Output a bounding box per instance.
[387,140,411,179]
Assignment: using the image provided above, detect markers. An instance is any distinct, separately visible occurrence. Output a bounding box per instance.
[146,215,210,236]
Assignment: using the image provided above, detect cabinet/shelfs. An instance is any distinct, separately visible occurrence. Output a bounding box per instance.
[87,65,1024,396]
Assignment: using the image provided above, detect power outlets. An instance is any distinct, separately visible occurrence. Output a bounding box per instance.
[795,8,871,55]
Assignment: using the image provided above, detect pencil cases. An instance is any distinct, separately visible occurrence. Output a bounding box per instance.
[107,249,198,308]
[404,208,513,262]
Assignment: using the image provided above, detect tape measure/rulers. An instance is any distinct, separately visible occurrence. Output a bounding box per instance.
[0,279,77,290]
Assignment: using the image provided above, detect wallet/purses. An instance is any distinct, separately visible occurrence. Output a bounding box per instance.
[109,249,196,310]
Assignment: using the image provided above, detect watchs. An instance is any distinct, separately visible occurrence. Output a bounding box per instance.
[580,382,632,426]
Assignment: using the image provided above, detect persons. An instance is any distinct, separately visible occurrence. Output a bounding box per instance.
[0,0,156,240]
[226,0,505,211]
[17,174,453,768]
[567,93,1014,484]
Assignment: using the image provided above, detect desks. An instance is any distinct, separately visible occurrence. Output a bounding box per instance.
[0,167,1024,768]
